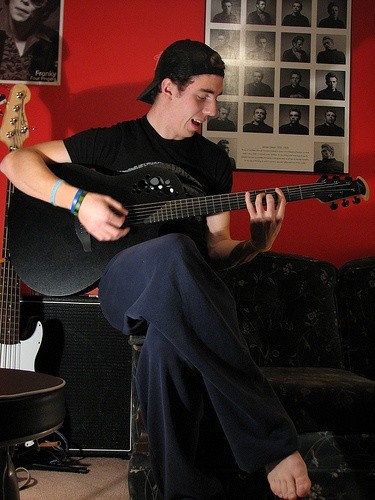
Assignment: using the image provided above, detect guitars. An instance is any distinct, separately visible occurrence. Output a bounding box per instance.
[0,81,44,373]
[8,160,367,298]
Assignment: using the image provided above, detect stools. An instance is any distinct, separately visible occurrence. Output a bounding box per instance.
[0,367,68,500]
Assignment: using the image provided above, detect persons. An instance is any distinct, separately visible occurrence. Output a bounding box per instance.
[213,0,273,25]
[314,143,344,173]
[213,31,237,58]
[281,72,310,98]
[0,39,311,500]
[248,34,273,61]
[281,1,309,26]
[208,106,235,131]
[217,139,236,169]
[315,110,344,136]
[245,70,274,97]
[243,105,274,133]
[0,0,57,80]
[317,2,346,29]
[317,36,346,64]
[278,109,309,135]
[282,35,309,63]
[314,73,346,100]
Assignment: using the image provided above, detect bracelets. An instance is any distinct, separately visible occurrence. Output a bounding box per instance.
[69,189,89,219]
[50,179,64,206]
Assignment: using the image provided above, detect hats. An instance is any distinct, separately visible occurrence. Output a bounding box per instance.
[137,39,226,104]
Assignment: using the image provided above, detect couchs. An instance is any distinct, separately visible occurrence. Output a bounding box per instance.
[129,251,375,500]
